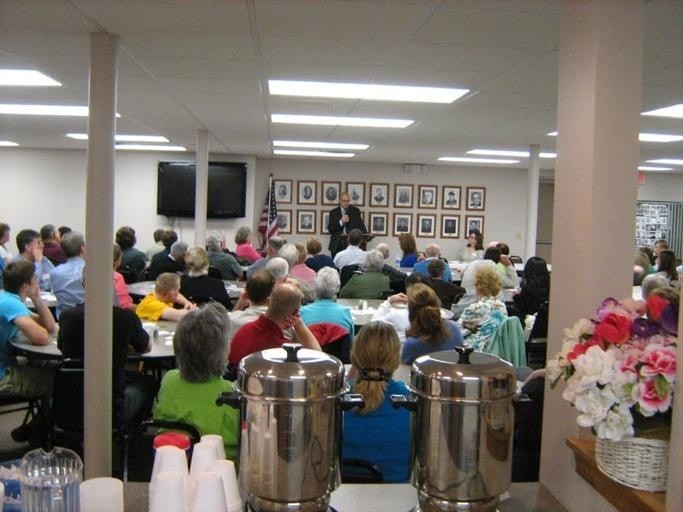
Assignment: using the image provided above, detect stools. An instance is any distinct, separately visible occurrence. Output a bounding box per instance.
[0,393,43,427]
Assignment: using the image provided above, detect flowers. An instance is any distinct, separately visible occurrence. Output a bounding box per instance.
[544,290,679,444]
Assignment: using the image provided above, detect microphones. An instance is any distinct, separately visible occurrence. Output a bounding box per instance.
[345,208,349,228]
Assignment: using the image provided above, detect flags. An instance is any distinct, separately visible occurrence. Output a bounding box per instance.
[259,178,280,249]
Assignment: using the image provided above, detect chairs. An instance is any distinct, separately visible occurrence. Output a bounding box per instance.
[117,418,201,483]
[339,458,384,484]
[307,323,351,364]
[40,357,137,482]
[446,256,547,371]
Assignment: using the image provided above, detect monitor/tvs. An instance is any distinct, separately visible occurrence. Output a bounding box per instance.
[157,161,246,218]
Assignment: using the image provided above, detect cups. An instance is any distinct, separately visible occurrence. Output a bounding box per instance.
[77,476,123,512]
[147,433,244,512]
[19,445,81,512]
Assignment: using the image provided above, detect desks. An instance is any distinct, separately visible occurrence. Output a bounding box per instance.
[10,313,213,379]
[337,297,456,329]
[22,277,257,310]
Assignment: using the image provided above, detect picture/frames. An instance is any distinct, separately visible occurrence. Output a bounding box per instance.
[272,178,485,239]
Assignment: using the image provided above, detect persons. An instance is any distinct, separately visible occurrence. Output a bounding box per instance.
[398,220,408,231]
[446,220,455,233]
[329,193,368,259]
[471,192,482,206]
[276,183,286,198]
[328,187,336,199]
[374,219,383,230]
[515,368,546,485]
[634,240,683,302]
[423,190,432,204]
[152,301,247,480]
[375,188,384,201]
[446,190,457,205]
[423,220,431,232]
[0,225,551,443]
[339,322,415,482]
[469,222,480,231]
[399,189,409,202]
[277,217,286,227]
[302,216,311,228]
[634,204,669,249]
[303,186,311,199]
[58,264,152,481]
[352,188,360,199]
